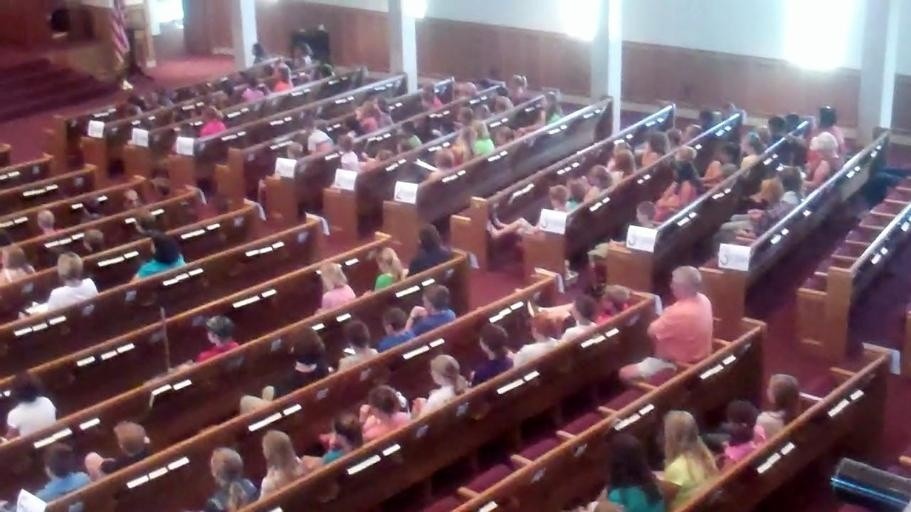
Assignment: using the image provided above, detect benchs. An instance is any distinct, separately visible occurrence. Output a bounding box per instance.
[0,164,98,213]
[0,203,261,322]
[161,73,408,195]
[597,116,815,294]
[449,104,677,273]
[239,282,657,512]
[699,128,890,346]
[0,176,147,247]
[78,65,320,179]
[659,394,823,512]
[0,232,392,440]
[385,97,614,248]
[211,77,455,201]
[0,151,52,192]
[521,110,746,281]
[126,66,367,179]
[0,218,322,380]
[260,85,501,222]
[35,57,282,159]
[0,185,199,274]
[39,269,559,511]
[320,96,546,237]
[0,249,470,505]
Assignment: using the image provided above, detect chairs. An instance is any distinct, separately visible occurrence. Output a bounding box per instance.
[832,243,870,260]
[680,338,737,430]
[796,271,851,357]
[897,179,911,187]
[710,317,768,419]
[860,212,897,230]
[847,226,885,245]
[421,490,473,512]
[456,454,532,512]
[870,199,908,216]
[636,363,696,447]
[509,430,587,505]
[815,256,859,278]
[0,142,13,166]
[798,368,860,464]
[886,188,911,202]
[557,407,621,507]
[832,347,891,443]
[599,384,661,483]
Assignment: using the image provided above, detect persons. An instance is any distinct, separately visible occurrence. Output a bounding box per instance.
[18,251,98,319]
[252,43,266,63]
[199,91,216,113]
[717,207,769,250]
[791,105,842,163]
[665,128,685,147]
[562,296,597,343]
[172,123,197,153]
[371,149,392,163]
[515,313,559,365]
[612,139,632,156]
[785,111,800,130]
[369,95,395,127]
[654,162,707,221]
[584,165,617,202]
[303,117,329,154]
[407,286,455,338]
[1,374,56,443]
[776,162,812,204]
[65,117,83,138]
[352,102,380,135]
[683,124,701,142]
[703,140,740,184]
[84,422,158,481]
[132,234,187,284]
[134,213,173,237]
[317,263,355,312]
[129,94,150,111]
[649,408,716,506]
[295,70,312,83]
[474,103,492,119]
[578,432,661,512]
[106,157,128,189]
[508,74,532,106]
[614,150,636,183]
[449,142,466,165]
[237,69,255,84]
[487,185,573,241]
[404,225,451,277]
[529,89,566,126]
[114,103,134,117]
[717,102,735,121]
[532,95,562,130]
[753,125,776,152]
[431,148,453,177]
[256,77,271,97]
[63,146,84,171]
[586,201,668,293]
[172,106,185,122]
[796,136,844,184]
[767,116,787,141]
[336,320,380,372]
[491,88,513,111]
[722,402,762,469]
[256,430,311,499]
[664,147,702,193]
[199,107,225,139]
[217,75,234,89]
[36,442,91,502]
[338,116,359,141]
[398,124,422,150]
[566,178,591,207]
[320,411,368,466]
[642,134,669,167]
[288,40,313,69]
[199,81,213,91]
[240,327,332,413]
[84,230,104,255]
[599,283,631,323]
[467,123,496,155]
[494,127,516,149]
[179,86,193,98]
[241,80,261,104]
[739,134,765,168]
[285,136,305,160]
[195,314,240,364]
[319,63,334,78]
[312,24,328,67]
[449,81,468,99]
[427,127,440,140]
[472,325,516,387]
[156,87,173,103]
[618,266,714,389]
[865,163,911,208]
[220,84,243,107]
[200,448,260,512]
[374,247,407,293]
[121,187,142,210]
[758,178,798,217]
[757,374,802,443]
[144,92,159,109]
[272,63,292,93]
[455,108,475,129]
[271,53,287,73]
[415,353,471,415]
[145,177,172,205]
[37,210,57,235]
[255,146,280,177]
[420,82,441,112]
[440,118,457,138]
[0,246,36,285]
[336,135,359,172]
[373,305,413,354]
[697,109,719,130]
[360,384,411,443]
[313,119,334,142]
[186,100,199,118]
